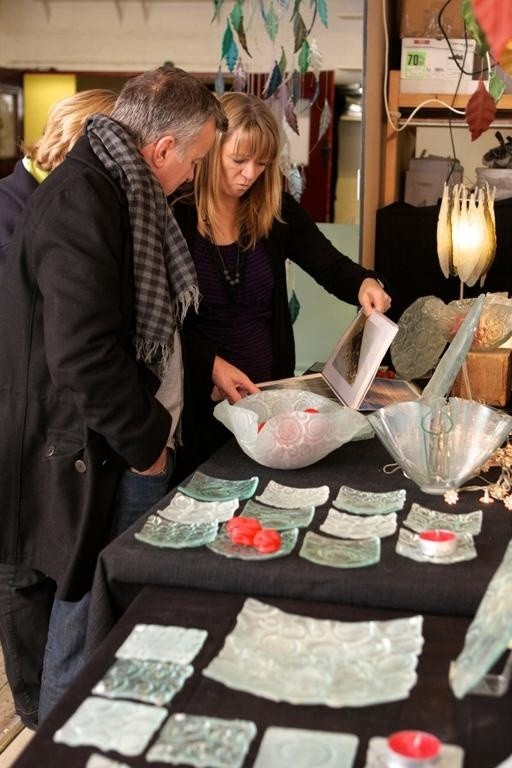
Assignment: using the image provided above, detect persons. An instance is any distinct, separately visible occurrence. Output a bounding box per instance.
[166,93,392,479]
[0,87,115,731]
[0,67,258,731]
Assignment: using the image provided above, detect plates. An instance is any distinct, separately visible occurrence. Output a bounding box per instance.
[255,478,331,510]
[236,498,314,530]
[254,724,359,768]
[135,515,218,551]
[206,519,299,563]
[178,472,257,503]
[384,728,441,768]
[403,503,484,535]
[298,531,382,568]
[199,598,426,705]
[54,697,166,753]
[364,736,464,768]
[94,658,195,705]
[156,493,239,524]
[144,715,257,768]
[318,507,398,540]
[421,529,459,557]
[332,485,407,515]
[116,620,206,663]
[394,528,480,566]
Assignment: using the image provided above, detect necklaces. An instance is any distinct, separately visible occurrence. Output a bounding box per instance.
[198,198,244,289]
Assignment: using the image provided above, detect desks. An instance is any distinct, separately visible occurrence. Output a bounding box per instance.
[93,431,512,584]
[10,588,512,763]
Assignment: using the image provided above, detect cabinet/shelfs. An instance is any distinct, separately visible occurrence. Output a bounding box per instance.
[362,3,511,269]
